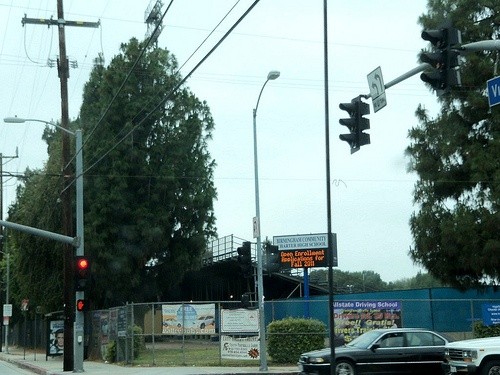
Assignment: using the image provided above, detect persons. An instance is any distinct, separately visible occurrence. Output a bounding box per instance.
[387,335,398,346]
[50,329,64,354]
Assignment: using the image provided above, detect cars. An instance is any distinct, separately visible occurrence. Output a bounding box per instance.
[441,335,500,375]
[296,327,453,375]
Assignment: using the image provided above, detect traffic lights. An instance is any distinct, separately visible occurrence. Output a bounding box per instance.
[267,243,281,274]
[237,242,251,261]
[420,26,463,97]
[75,254,88,292]
[338,100,361,154]
[359,100,371,148]
[77,299,85,311]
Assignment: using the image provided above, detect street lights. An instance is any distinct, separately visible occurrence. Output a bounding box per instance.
[3,115,85,375]
[250,68,281,374]
